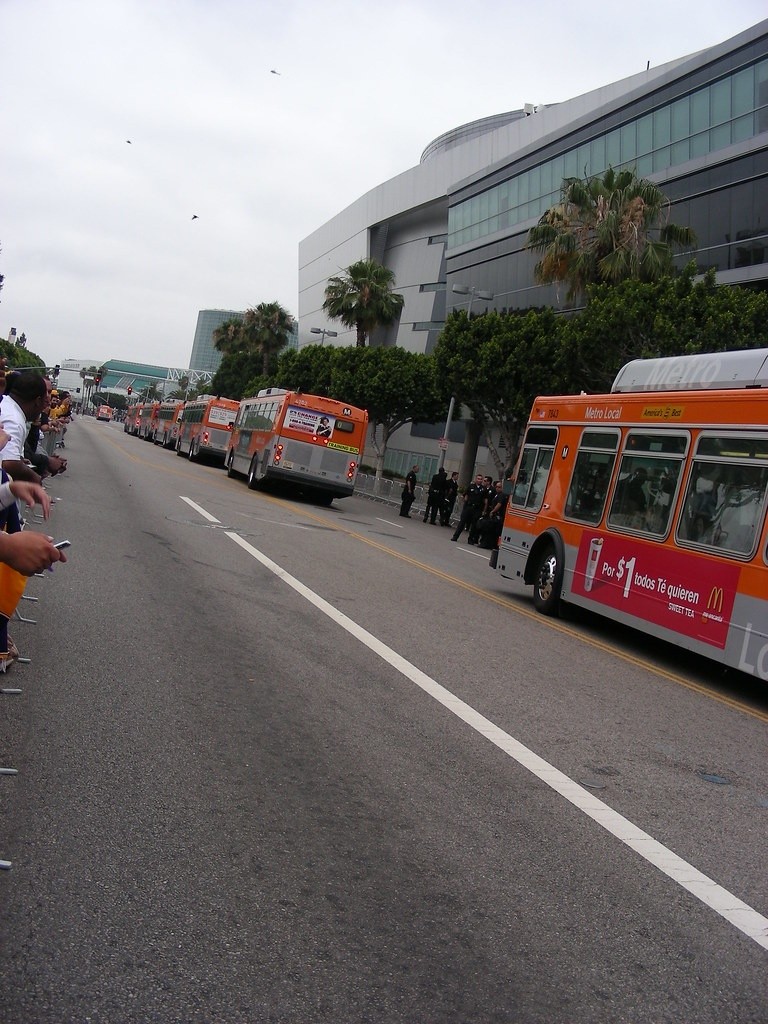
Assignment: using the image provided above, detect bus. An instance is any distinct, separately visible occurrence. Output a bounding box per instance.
[223,387,369,505]
[123,398,186,446]
[175,394,241,462]
[96,405,112,421]
[490,348,767,686]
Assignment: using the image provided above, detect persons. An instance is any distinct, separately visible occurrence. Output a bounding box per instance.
[450,475,507,550]
[316,416,332,437]
[423,467,459,527]
[399,465,419,518]
[0,357,74,670]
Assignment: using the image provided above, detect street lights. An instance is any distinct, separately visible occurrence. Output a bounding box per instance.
[311,327,337,347]
[427,284,494,473]
[144,384,154,399]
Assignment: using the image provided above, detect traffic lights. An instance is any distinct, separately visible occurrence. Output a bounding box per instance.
[128,387,132,395]
[95,378,100,386]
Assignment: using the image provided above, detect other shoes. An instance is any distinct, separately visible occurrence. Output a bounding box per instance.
[451,537,457,542]
[429,522,436,525]
[445,523,452,528]
[399,514,412,518]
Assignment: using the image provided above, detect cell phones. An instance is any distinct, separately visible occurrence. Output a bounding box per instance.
[53,539,71,550]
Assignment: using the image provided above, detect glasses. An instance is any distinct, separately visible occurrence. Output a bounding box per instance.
[484,480,487,482]
